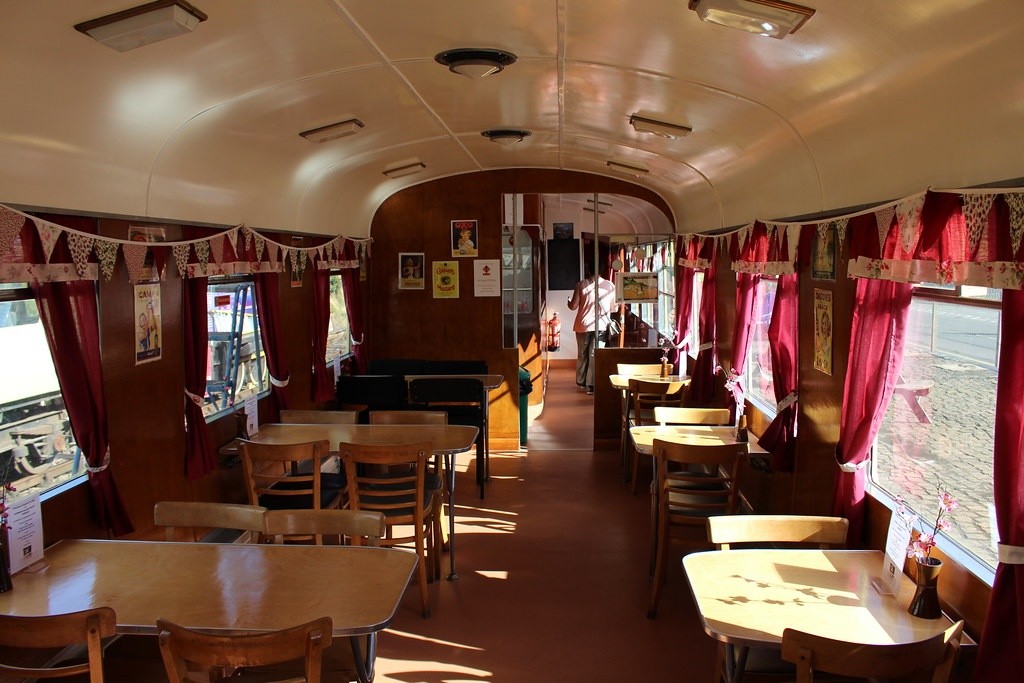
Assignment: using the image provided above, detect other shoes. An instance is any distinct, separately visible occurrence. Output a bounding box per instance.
[587,385,594,394]
[580,383,586,388]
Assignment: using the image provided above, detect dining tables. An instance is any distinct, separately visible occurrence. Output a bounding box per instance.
[681,549,979,683]
[608,363,771,500]
[218,424,479,582]
[0,536,420,682]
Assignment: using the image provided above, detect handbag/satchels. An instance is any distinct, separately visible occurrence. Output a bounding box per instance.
[608,319,622,336]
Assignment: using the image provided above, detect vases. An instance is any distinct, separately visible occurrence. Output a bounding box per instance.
[235,414,250,444]
[907,555,943,621]
[0,537,14,593]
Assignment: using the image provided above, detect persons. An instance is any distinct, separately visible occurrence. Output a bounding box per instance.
[139,300,158,351]
[458,230,474,250]
[403,256,422,279]
[816,311,831,371]
[567,256,618,396]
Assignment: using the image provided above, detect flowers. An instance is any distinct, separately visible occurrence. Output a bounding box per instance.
[0,480,16,531]
[893,482,955,566]
[222,375,241,416]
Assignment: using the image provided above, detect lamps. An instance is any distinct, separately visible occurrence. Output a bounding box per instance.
[73,0,208,53]
[383,162,428,180]
[298,119,364,145]
[629,116,693,140]
[434,47,519,82]
[689,0,815,41]
[606,160,649,178]
[481,130,533,146]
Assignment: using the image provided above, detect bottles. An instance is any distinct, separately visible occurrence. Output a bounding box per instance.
[548,311,561,351]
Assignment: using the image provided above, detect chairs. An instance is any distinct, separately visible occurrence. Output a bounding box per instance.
[244,508,386,670]
[780,618,964,683]
[235,437,347,549]
[364,411,452,553]
[270,409,359,501]
[332,355,505,485]
[0,606,168,683]
[102,500,267,654]
[641,438,750,619]
[339,439,438,619]
[154,617,357,683]
[707,514,849,683]
